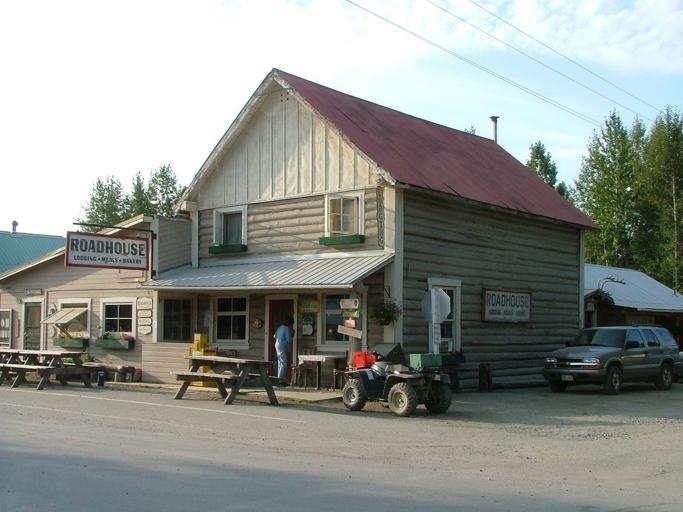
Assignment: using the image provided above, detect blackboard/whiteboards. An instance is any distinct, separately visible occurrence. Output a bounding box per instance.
[342,350,452,416]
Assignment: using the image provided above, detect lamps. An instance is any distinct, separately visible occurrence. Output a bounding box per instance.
[331,350,348,391]
[292,346,317,391]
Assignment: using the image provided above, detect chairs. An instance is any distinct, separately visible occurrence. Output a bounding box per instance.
[277,382,290,388]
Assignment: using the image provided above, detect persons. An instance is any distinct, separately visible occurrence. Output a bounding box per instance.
[272,315,297,387]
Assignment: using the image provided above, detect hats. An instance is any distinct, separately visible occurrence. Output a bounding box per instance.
[542,324,681,395]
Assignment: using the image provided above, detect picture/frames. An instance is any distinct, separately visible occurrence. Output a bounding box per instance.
[298,353,344,391]
[173,355,284,406]
[0,348,92,390]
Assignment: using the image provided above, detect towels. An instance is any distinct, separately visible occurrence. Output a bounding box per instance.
[479,364,492,393]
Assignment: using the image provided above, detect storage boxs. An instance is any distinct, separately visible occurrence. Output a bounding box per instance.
[0,362,58,391]
[44,360,105,389]
[237,370,287,394]
[174,370,238,405]
